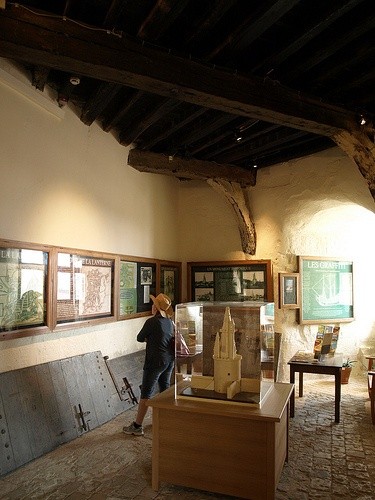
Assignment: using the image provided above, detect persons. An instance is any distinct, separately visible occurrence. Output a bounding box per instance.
[143,270,151,282]
[123,293,175,436]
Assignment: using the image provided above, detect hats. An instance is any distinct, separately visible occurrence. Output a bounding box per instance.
[150,293,171,318]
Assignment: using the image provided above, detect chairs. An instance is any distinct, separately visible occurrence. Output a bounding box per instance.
[366,357,375,425]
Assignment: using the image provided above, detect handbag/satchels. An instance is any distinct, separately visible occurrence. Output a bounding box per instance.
[171,321,190,358]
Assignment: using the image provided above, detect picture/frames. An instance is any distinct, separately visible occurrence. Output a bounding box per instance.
[280,273,301,309]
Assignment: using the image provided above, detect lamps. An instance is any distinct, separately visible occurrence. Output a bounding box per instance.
[233,131,243,142]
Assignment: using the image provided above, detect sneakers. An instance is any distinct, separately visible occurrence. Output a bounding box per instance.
[122,420,143,435]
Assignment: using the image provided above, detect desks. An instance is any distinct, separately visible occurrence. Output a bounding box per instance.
[287,350,343,423]
[177,345,202,375]
